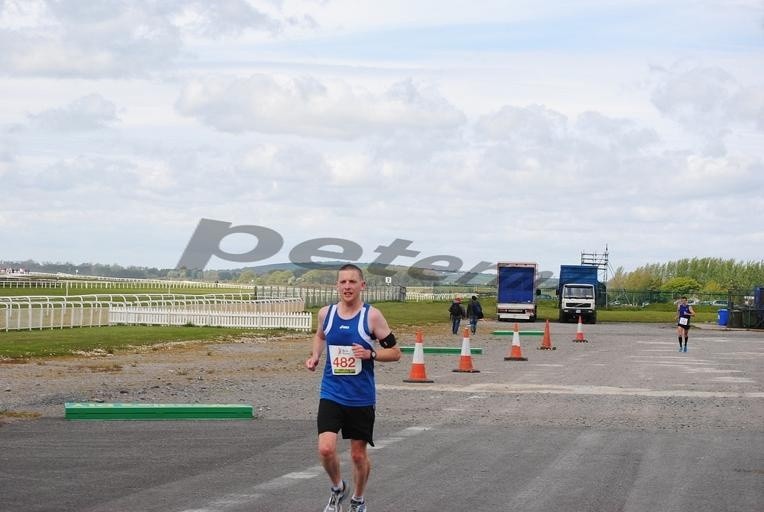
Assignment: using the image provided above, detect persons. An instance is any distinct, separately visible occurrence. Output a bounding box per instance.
[452,298,465,335]
[672,296,695,352]
[467,296,482,335]
[305,264,401,512]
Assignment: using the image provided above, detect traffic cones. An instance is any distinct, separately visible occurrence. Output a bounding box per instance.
[537,319,556,350]
[572,315,587,343]
[451,327,480,373]
[402,329,433,383]
[503,322,528,361]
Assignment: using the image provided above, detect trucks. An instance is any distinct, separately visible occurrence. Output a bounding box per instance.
[744,295,754,307]
[555,264,597,324]
[495,261,540,322]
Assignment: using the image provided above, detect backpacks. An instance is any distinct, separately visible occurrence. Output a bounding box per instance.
[472,301,482,314]
[450,303,461,316]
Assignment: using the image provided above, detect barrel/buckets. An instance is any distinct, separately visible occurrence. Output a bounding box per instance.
[730,310,743,328]
[753,287,764,309]
[717,309,729,325]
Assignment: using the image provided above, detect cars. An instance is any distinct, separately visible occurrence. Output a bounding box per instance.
[701,300,712,306]
[607,297,654,307]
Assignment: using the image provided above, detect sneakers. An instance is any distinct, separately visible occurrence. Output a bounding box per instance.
[324,479,350,512]
[680,347,687,352]
[347,497,367,512]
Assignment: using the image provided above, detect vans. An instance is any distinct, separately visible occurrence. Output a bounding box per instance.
[711,300,739,308]
[673,297,700,306]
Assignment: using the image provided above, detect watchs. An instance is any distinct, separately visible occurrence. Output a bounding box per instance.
[370,350,377,360]
